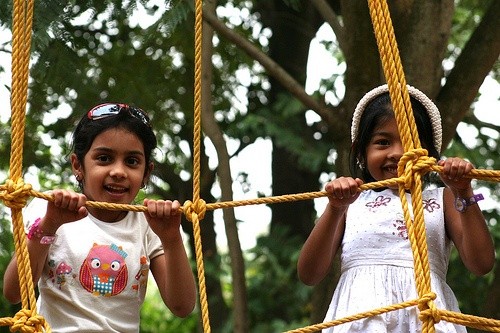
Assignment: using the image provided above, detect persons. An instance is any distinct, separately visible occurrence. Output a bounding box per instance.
[298,83,495,333]
[4,102,197,333]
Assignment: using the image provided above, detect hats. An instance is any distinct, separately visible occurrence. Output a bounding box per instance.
[351,84,444,176]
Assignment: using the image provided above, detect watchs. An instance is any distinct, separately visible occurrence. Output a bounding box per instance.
[455,193,483,214]
[28,219,56,245]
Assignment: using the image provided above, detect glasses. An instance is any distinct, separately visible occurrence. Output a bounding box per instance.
[86,103,153,126]
